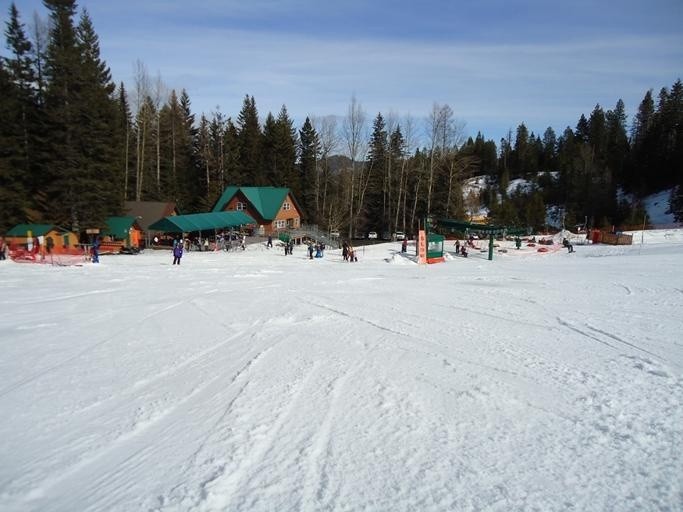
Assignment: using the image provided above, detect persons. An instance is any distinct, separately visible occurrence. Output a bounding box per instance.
[29,236,40,255]
[461,245,468,258]
[563,237,575,253]
[266,233,273,247]
[515,235,521,249]
[153,229,246,265]
[0,235,7,260]
[92,235,100,264]
[470,235,474,243]
[342,241,355,263]
[284,236,294,256]
[454,239,461,254]
[583,227,591,245]
[401,235,408,253]
[308,239,325,261]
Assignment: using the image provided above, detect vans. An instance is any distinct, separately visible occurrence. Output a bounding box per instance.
[330,229,339,237]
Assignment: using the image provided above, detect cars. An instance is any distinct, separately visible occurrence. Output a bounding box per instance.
[395,231,405,239]
[368,231,377,239]
